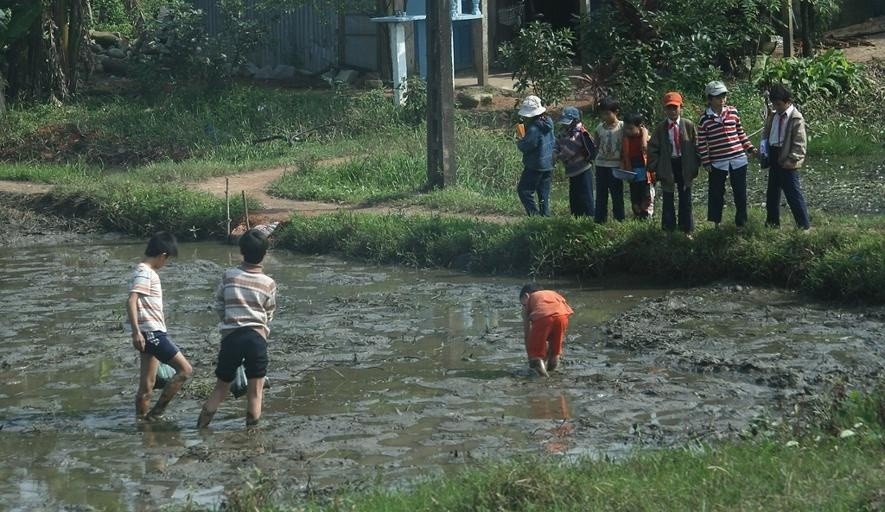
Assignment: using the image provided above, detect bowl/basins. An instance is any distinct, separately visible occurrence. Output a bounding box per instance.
[610,167,637,182]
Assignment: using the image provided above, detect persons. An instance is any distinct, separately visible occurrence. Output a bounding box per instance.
[198,229,277,428]
[554,105,595,215]
[620,111,658,219]
[593,96,625,223]
[759,82,812,233]
[696,81,758,233]
[517,95,556,217]
[521,283,574,380]
[648,91,701,234]
[126,231,192,422]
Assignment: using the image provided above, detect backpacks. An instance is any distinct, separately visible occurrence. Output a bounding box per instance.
[569,128,596,163]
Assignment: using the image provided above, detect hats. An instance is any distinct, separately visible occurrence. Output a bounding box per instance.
[705,81,728,97]
[556,105,580,125]
[663,92,682,108]
[517,96,546,118]
